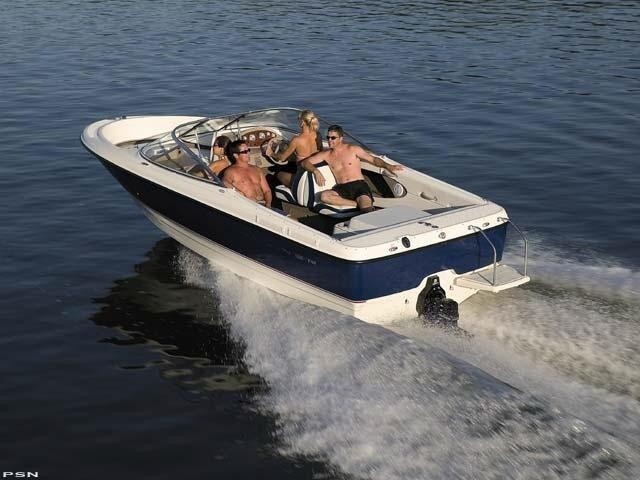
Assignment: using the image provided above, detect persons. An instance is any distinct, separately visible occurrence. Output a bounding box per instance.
[266,108,323,191]
[188,136,233,181]
[218,139,298,223]
[299,125,406,214]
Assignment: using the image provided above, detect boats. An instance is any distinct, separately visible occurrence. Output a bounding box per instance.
[80,105,530,341]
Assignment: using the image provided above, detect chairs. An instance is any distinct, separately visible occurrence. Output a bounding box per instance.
[274,160,362,219]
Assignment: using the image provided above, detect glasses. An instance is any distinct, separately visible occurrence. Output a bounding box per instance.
[326,136,340,140]
[237,148,251,154]
